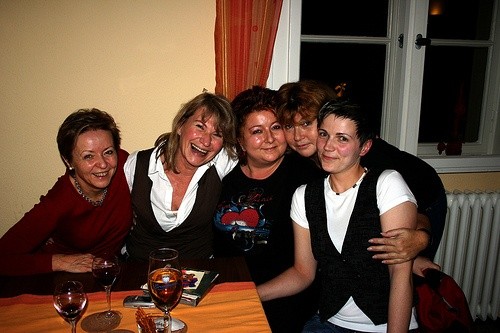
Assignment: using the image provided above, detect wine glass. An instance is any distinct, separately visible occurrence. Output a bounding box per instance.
[92,253,123,323]
[147,249,183,333]
[53,281,88,333]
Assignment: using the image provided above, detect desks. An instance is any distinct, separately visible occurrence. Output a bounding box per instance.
[0,281,273,333]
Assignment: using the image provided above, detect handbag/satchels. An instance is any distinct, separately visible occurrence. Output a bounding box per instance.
[412,267,476,333]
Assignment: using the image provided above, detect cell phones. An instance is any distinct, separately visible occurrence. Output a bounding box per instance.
[123,295,156,309]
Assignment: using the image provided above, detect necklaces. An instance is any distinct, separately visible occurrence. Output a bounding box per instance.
[74,175,107,205]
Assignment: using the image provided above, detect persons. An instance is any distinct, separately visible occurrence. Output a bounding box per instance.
[0,108,133,299]
[123,93,237,289]
[275,81,447,276]
[256,96,418,333]
[214,86,430,333]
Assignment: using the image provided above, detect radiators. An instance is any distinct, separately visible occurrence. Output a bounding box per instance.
[432,189,500,321]
